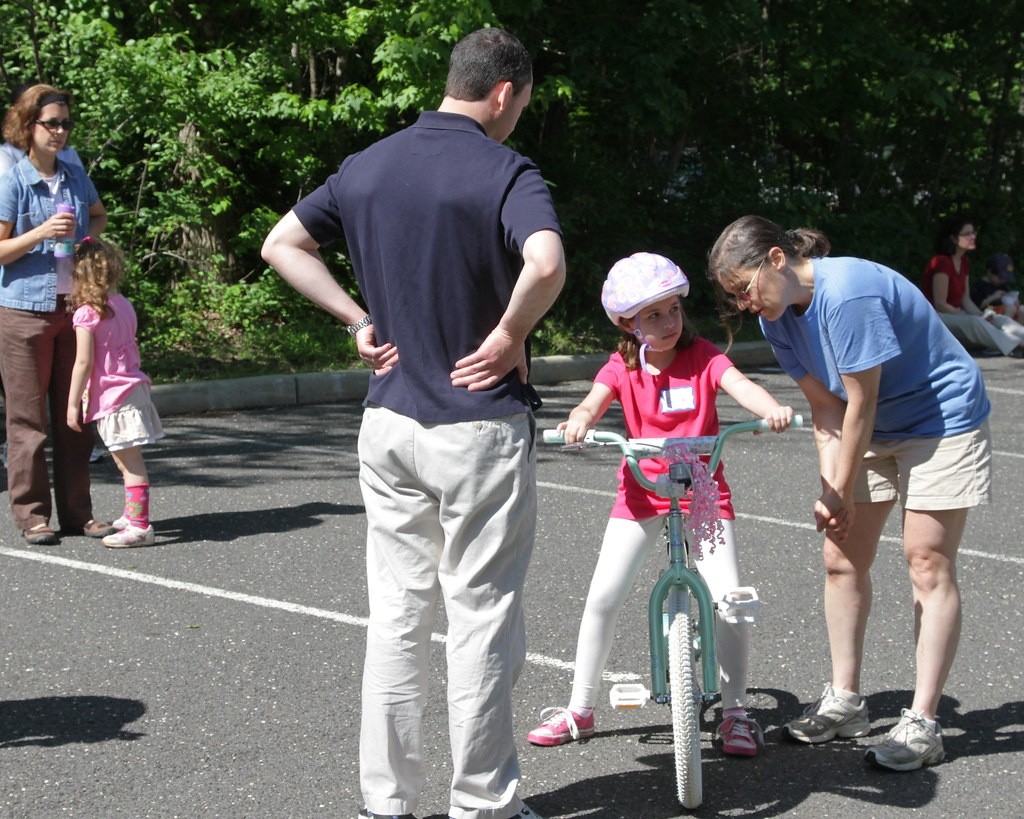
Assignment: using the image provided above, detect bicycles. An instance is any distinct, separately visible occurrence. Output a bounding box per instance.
[542,415,807,809]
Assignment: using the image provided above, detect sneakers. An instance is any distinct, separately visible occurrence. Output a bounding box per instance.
[782,682,870,743]
[527,707,595,746]
[717,714,765,756]
[864,707,945,771]
[102,524,155,547]
[112,515,130,529]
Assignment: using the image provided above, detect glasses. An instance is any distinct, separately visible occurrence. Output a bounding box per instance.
[35,120,75,130]
[727,259,765,305]
[954,231,977,239]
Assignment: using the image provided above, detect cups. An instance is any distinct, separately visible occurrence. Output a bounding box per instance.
[55,204,75,258]
[1001,291,1021,318]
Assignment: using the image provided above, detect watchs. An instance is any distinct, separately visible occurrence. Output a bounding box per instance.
[347,314,373,336]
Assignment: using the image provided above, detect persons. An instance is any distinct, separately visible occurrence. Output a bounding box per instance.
[259,29,567,819]
[0,82,158,547]
[710,214,990,772]
[526,252,793,757]
[922,215,1024,356]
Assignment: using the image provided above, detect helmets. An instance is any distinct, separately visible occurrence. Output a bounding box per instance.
[601,252,690,326]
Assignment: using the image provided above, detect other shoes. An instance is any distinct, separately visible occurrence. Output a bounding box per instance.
[75,519,116,536]
[24,524,58,543]
[508,804,543,819]
[1014,347,1024,358]
[358,809,417,819]
